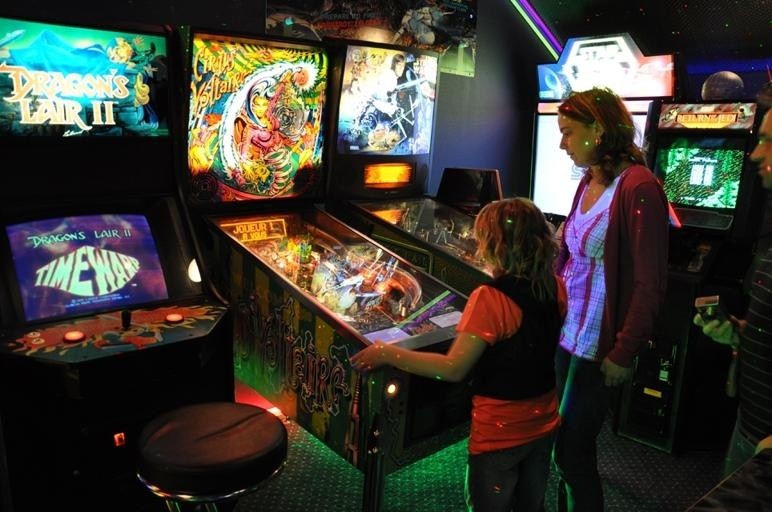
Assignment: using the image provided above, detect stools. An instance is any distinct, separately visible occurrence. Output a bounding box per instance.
[135,401,289,511]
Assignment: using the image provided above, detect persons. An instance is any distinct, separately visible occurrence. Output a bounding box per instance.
[694,108,771,482]
[350,196,568,512]
[554,89,672,512]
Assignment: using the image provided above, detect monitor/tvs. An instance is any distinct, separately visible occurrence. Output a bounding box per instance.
[647,140,746,214]
[186,28,331,205]
[0,195,212,326]
[529,97,649,223]
[336,39,441,161]
[0,14,173,143]
